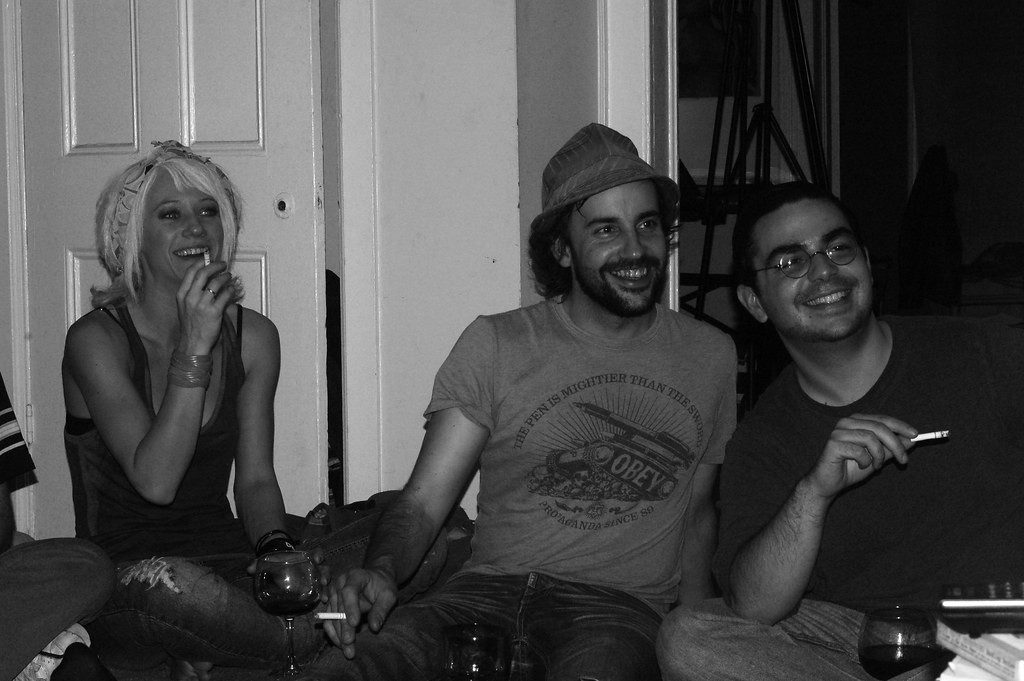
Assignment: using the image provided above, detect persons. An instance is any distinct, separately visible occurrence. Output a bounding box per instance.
[299,124,738,681]
[656,181,1024,681]
[63,141,450,681]
[0,372,116,681]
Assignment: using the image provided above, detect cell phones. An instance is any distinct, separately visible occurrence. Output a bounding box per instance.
[936,581,1023,611]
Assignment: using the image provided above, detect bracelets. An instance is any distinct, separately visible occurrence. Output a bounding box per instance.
[167,349,212,387]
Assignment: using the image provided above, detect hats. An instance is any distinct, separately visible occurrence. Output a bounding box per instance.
[540,123,680,213]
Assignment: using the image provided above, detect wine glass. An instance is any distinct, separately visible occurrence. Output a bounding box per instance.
[254,551,323,681]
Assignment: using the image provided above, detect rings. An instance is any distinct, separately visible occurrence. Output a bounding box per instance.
[205,287,217,295]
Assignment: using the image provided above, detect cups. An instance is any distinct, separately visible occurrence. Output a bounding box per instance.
[440,623,512,681]
[857,607,939,681]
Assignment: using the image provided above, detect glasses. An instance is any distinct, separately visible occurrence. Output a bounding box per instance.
[745,232,860,284]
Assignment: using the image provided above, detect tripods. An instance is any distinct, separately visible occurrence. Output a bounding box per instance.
[694,0,831,409]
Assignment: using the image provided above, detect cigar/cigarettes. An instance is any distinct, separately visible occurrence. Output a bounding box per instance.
[204,250,210,266]
[910,430,951,443]
[315,612,347,620]
